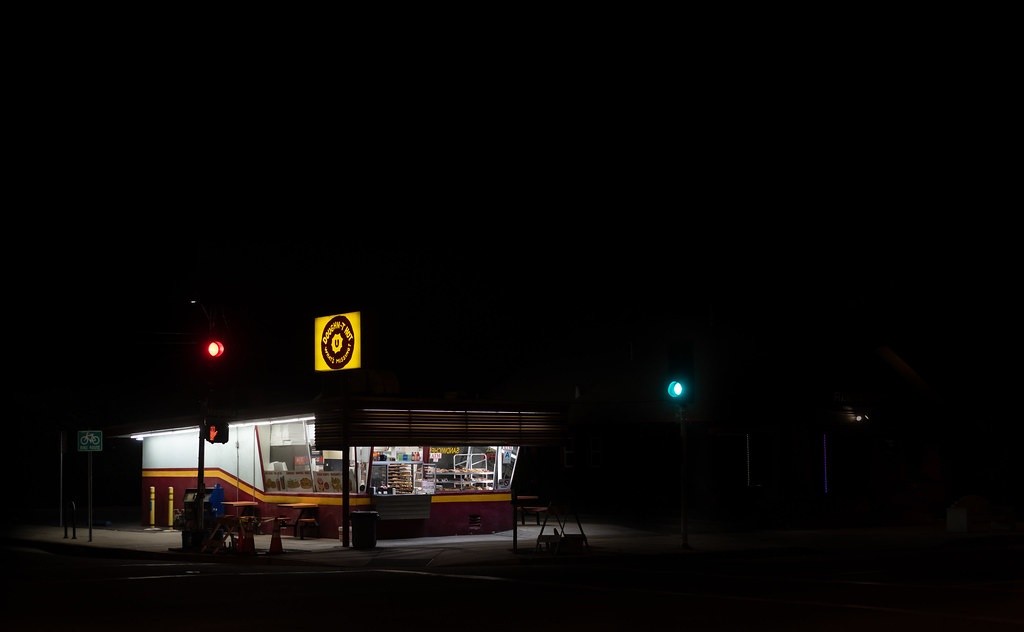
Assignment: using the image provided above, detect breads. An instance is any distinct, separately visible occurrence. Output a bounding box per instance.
[388,464,412,494]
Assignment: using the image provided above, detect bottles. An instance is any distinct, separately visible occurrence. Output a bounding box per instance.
[411,452,420,462]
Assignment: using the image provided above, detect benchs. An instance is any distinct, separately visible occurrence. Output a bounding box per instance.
[517,506,548,525]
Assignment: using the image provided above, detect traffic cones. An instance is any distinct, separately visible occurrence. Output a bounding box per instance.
[243,517,258,554]
[268,517,287,554]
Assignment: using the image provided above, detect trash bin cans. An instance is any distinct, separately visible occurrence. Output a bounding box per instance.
[351,510,378,548]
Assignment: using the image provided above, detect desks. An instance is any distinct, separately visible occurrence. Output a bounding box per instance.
[516,496,540,525]
[279,503,318,525]
[221,501,258,516]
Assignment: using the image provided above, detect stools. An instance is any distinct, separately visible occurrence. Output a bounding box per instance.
[218,514,325,540]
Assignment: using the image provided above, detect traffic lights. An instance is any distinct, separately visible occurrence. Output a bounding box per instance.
[205,340,225,358]
[666,342,694,403]
[206,418,228,443]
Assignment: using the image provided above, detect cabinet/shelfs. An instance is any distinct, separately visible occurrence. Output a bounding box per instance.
[371,461,496,491]
